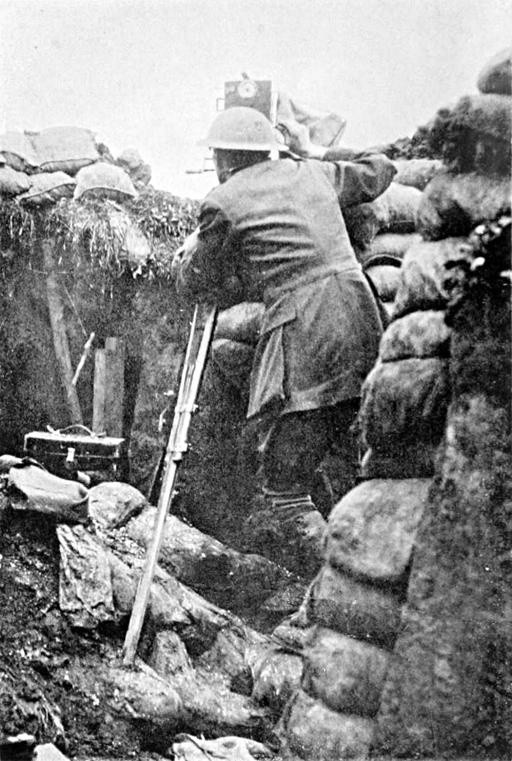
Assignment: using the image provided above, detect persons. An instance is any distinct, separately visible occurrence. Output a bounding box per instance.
[170,106,397,612]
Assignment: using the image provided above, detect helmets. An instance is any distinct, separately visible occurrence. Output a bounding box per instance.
[198,107,289,153]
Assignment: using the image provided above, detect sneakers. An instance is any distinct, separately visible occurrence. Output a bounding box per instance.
[259,576,309,615]
